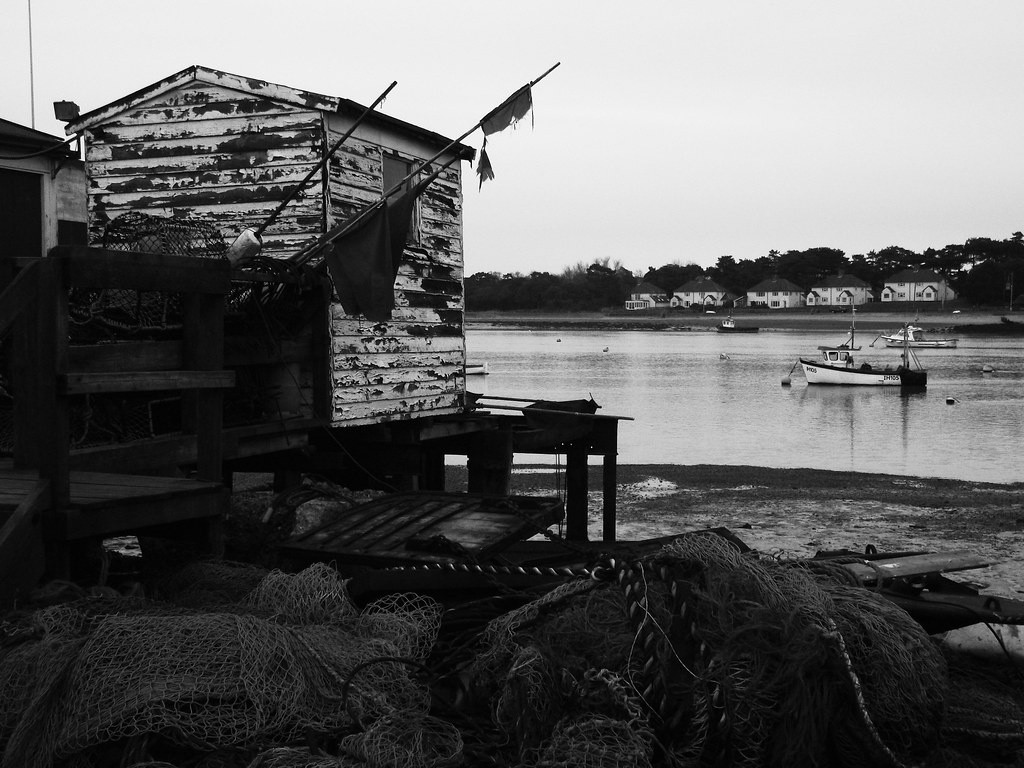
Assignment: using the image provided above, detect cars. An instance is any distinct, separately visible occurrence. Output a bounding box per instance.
[827,305,847,313]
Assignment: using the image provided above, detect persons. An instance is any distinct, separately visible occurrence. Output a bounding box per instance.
[901,354,909,368]
[845,352,854,368]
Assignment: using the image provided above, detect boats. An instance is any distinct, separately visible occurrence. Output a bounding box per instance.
[717,310,759,333]
[798,296,901,387]
[881,323,957,348]
[899,320,928,395]
[151,472,1024,638]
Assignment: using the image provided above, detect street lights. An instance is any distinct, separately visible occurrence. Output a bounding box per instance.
[1005,271,1013,311]
